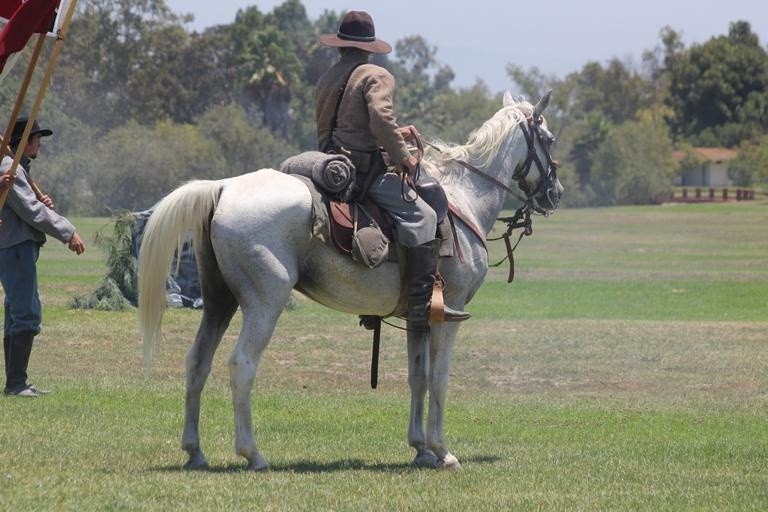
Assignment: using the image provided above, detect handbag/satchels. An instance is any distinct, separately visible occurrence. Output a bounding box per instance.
[350,223,390,269]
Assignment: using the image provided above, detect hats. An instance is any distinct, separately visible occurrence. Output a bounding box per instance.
[318,9,394,56]
[7,116,54,143]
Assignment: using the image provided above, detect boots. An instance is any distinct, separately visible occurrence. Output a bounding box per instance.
[3,332,51,395]
[2,326,39,398]
[402,235,472,327]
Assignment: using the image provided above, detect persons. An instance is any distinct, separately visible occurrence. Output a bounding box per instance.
[313,8,474,325]
[0,167,17,185]
[0,116,83,397]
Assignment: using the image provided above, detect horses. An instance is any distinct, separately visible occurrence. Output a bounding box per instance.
[136,87,566,474]
